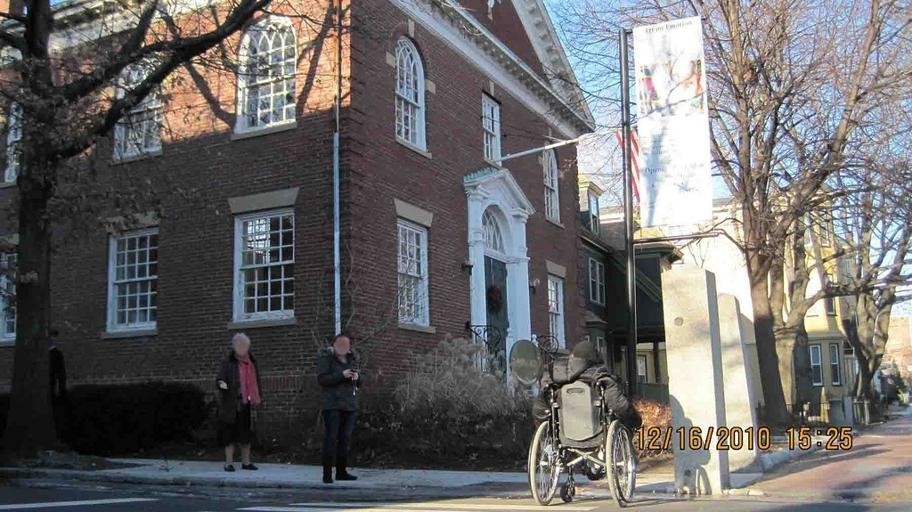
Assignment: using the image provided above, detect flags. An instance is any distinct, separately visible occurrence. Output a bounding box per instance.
[616,125,641,202]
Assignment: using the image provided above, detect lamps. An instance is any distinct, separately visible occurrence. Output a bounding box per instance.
[460,261,474,275]
[529,278,541,294]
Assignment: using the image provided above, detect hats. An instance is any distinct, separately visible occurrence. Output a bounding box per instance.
[574,341,604,363]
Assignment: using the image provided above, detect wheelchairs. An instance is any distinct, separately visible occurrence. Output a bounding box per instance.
[527,379,638,509]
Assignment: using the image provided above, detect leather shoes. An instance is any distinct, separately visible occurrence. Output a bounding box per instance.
[243,464,257,471]
[223,464,234,472]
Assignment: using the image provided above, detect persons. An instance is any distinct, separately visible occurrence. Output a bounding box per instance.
[540,338,640,480]
[315,332,364,481]
[47,333,69,439]
[215,333,260,468]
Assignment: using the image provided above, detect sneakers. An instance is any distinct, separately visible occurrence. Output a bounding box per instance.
[337,472,356,480]
